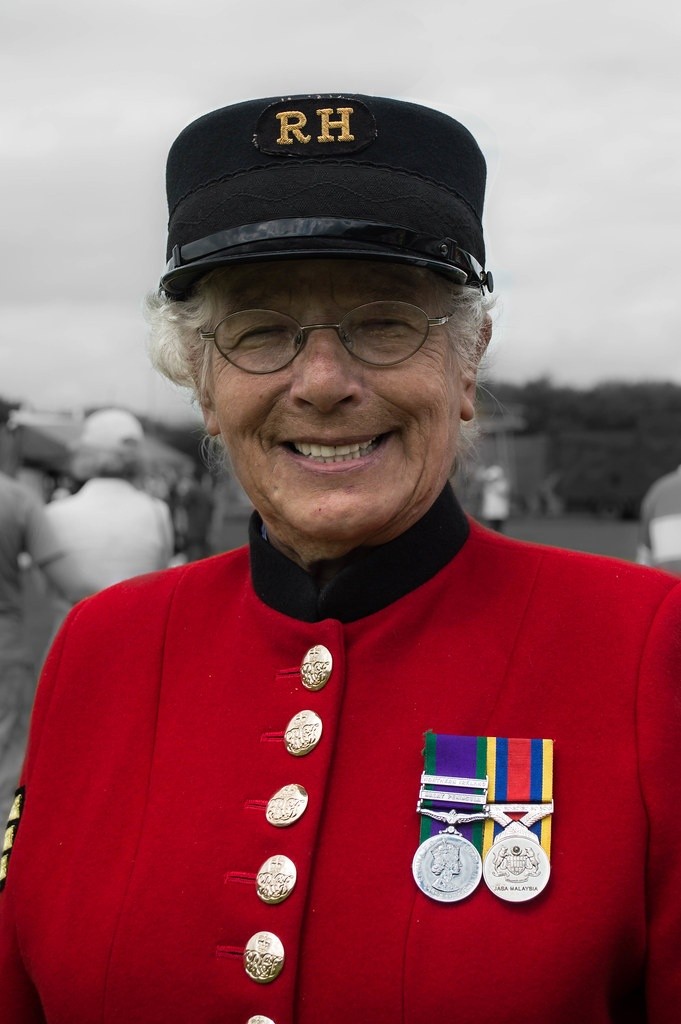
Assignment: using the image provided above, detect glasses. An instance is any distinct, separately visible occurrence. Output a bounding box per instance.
[198,301,456,374]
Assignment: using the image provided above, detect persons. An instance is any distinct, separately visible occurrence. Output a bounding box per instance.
[480,464,511,533]
[45,407,175,590]
[0,93,681,1024]
[0,470,99,847]
[160,465,213,559]
[636,463,681,574]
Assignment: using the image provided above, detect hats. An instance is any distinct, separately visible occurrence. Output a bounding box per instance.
[157,93,493,300]
[87,408,145,455]
